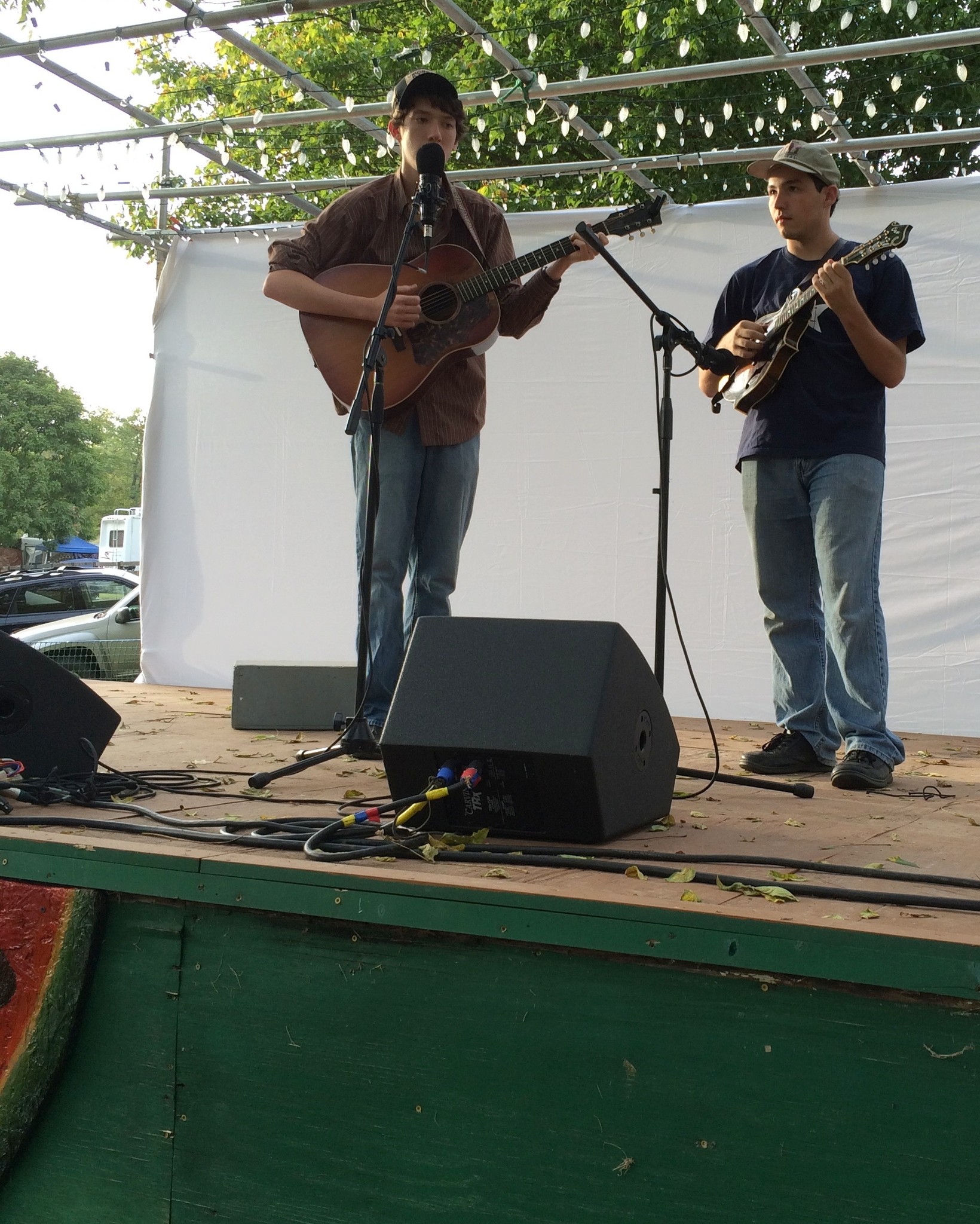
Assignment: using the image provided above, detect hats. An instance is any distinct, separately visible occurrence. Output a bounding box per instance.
[747,140,840,201]
[392,70,457,124]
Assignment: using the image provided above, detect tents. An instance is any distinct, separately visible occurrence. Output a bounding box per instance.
[30,534,99,573]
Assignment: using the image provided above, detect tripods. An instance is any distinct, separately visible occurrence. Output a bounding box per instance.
[247,189,429,787]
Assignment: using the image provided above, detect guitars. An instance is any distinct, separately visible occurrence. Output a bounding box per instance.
[717,218,913,413]
[298,191,668,412]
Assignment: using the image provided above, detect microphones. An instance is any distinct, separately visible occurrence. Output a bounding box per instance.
[416,143,446,250]
[0,787,41,805]
[695,338,737,376]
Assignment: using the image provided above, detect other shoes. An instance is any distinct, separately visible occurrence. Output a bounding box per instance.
[369,724,383,758]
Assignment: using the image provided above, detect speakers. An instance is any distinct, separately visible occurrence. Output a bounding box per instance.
[377,616,680,845]
[0,632,121,780]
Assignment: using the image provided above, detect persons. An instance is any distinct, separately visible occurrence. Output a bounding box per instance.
[699,140,927,791]
[261,70,609,758]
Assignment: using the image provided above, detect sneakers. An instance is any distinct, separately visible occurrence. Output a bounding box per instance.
[831,749,894,788]
[740,729,835,774]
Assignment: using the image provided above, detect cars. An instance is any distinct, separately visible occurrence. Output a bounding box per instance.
[0,557,141,682]
